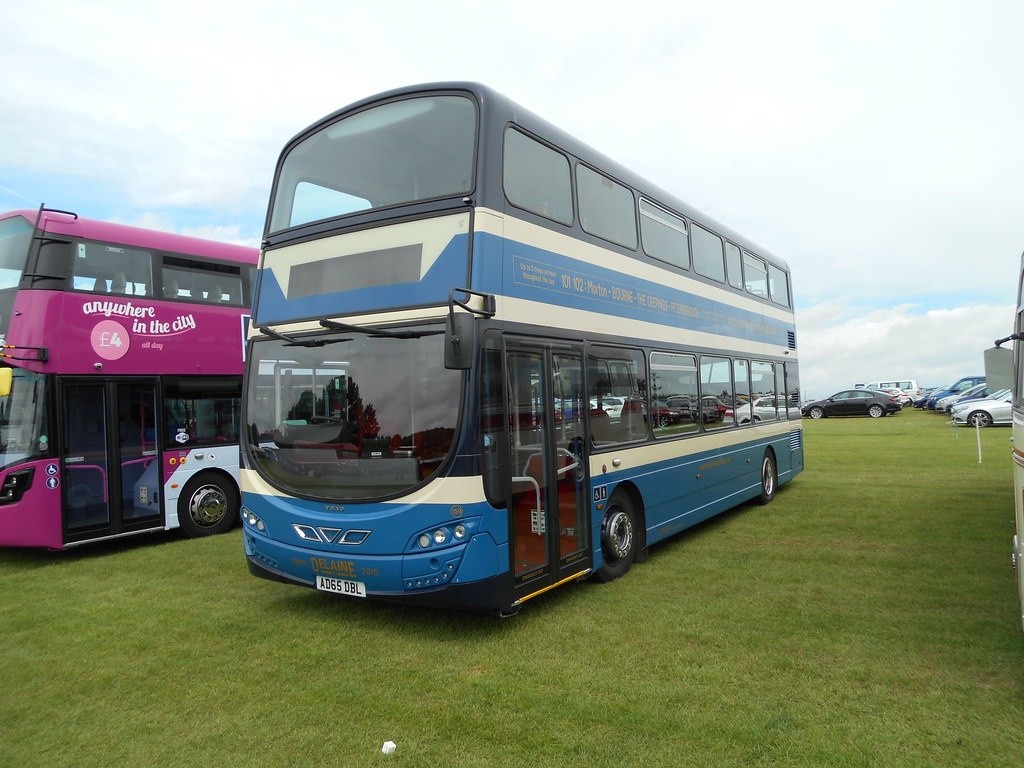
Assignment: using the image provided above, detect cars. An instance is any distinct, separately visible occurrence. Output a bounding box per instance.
[915,388,935,409]
[875,389,910,407]
[801,388,903,419]
[935,384,1013,428]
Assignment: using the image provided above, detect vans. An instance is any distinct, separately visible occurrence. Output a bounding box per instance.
[866,381,920,406]
[927,377,986,411]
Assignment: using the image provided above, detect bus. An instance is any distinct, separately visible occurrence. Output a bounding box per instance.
[237,82,805,619]
[0,203,261,556]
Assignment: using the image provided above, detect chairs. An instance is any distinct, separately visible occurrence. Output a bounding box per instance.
[92,272,240,304]
[532,401,653,450]
